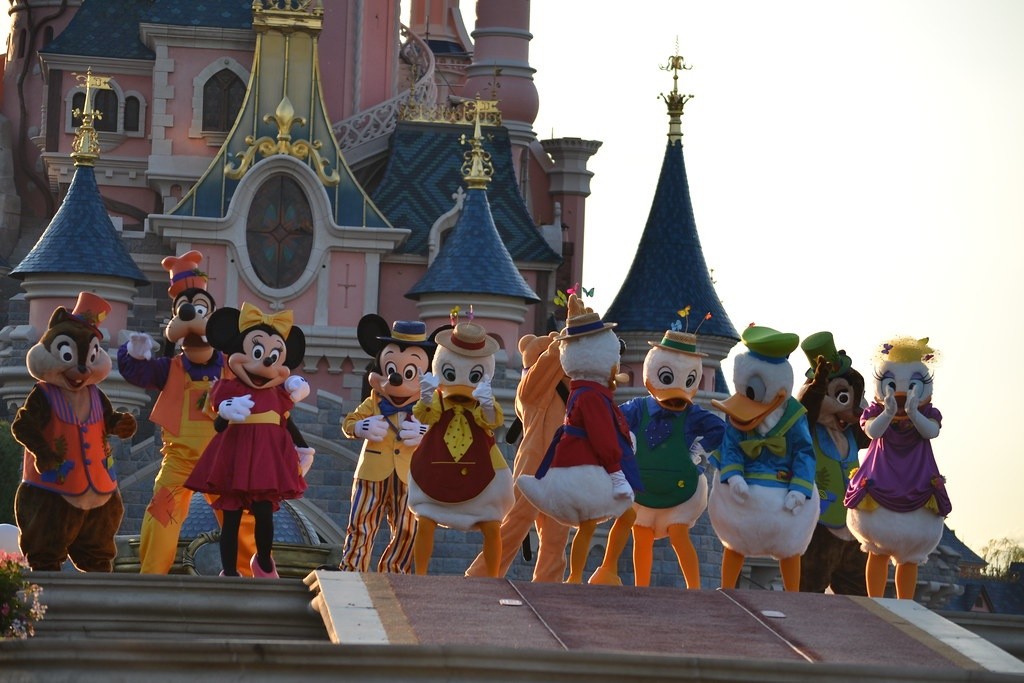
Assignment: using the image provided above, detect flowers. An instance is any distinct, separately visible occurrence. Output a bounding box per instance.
[0,548,47,639]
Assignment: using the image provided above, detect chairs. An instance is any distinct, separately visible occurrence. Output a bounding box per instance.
[183,529,225,577]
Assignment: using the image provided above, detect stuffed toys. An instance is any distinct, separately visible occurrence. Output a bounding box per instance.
[11,292,137,573]
[798,331,874,596]
[338,314,454,574]
[463,329,578,583]
[710,325,821,593]
[619,330,728,588]
[515,285,644,583]
[843,340,952,599]
[184,302,316,579]
[407,306,516,576]
[118,251,310,577]
